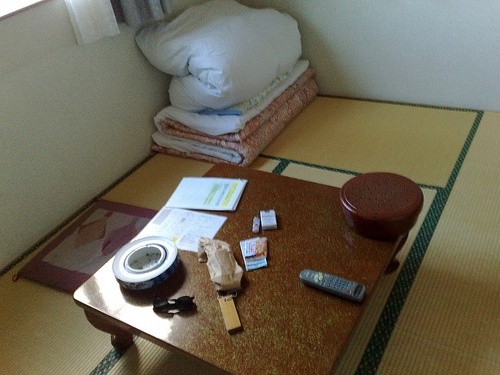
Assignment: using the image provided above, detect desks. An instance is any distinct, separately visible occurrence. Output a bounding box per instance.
[73,162,410,375]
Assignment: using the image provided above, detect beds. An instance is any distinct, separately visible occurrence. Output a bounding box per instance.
[0,94,500,375]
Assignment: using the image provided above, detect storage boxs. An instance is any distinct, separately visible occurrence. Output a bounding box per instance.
[260,210,278,231]
[339,172,424,241]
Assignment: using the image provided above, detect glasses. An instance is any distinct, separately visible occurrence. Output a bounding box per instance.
[153,296,195,314]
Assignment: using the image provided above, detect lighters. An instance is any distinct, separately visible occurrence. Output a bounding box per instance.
[252,215,260,233]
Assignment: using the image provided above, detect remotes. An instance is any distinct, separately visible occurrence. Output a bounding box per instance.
[299,269,366,303]
